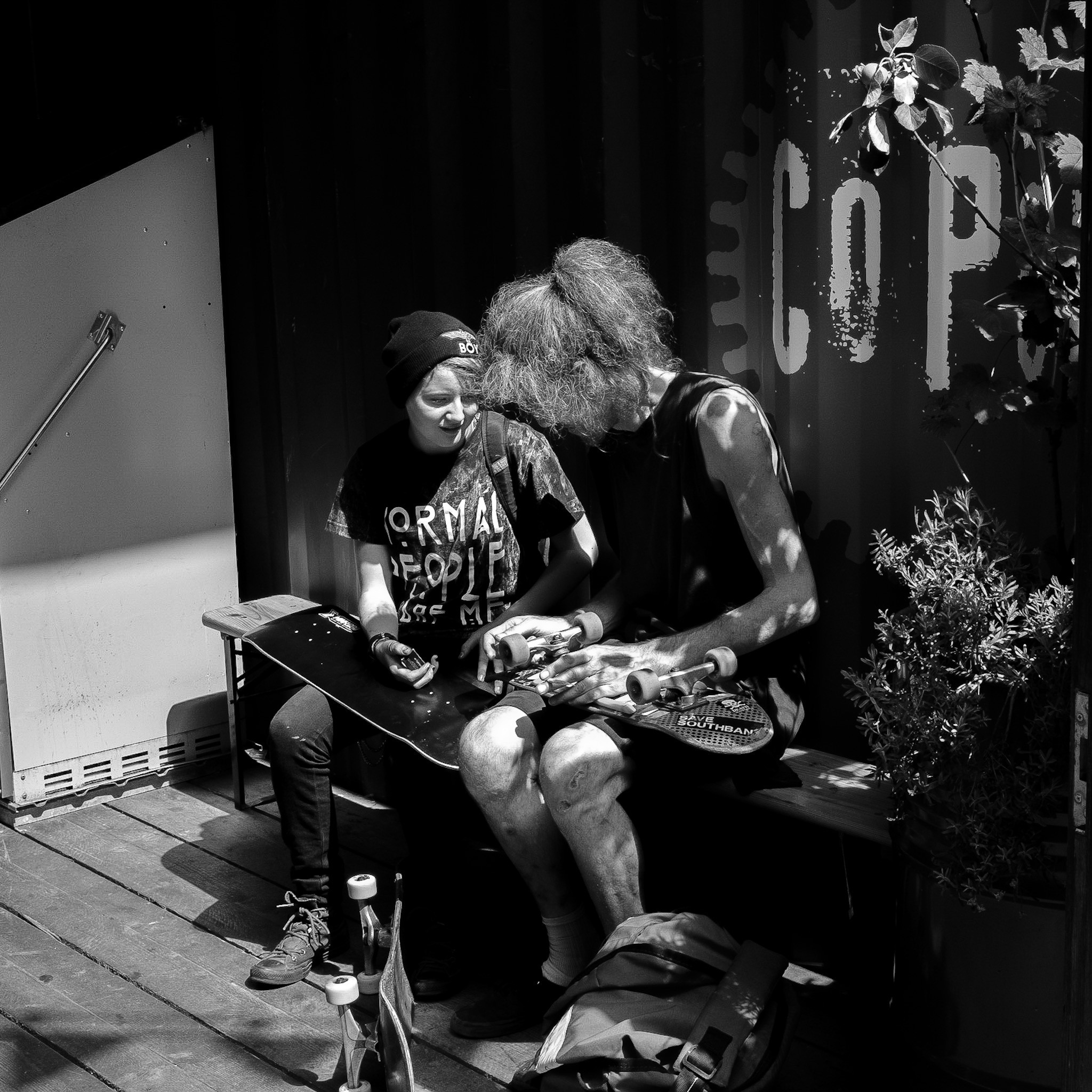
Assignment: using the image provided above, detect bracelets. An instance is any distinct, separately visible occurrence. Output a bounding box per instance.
[367,632,397,656]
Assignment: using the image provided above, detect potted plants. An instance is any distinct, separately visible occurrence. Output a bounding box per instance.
[837,438,1091,1092]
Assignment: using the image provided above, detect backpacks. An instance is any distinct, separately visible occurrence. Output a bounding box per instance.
[505,910,835,1092]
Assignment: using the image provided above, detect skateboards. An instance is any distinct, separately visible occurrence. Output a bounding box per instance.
[325,871,419,1092]
[242,602,508,772]
[457,609,774,756]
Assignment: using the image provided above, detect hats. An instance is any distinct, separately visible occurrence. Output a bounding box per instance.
[380,309,483,394]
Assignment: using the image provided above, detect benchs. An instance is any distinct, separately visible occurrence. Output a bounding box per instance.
[203,594,910,1026]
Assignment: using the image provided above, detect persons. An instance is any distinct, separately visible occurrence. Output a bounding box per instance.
[248,311,599,987]
[449,236,820,1042]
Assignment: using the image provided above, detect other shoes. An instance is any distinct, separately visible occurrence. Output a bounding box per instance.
[450,960,568,1040]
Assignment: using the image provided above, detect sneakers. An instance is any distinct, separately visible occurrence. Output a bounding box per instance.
[414,942,455,996]
[248,902,337,985]
